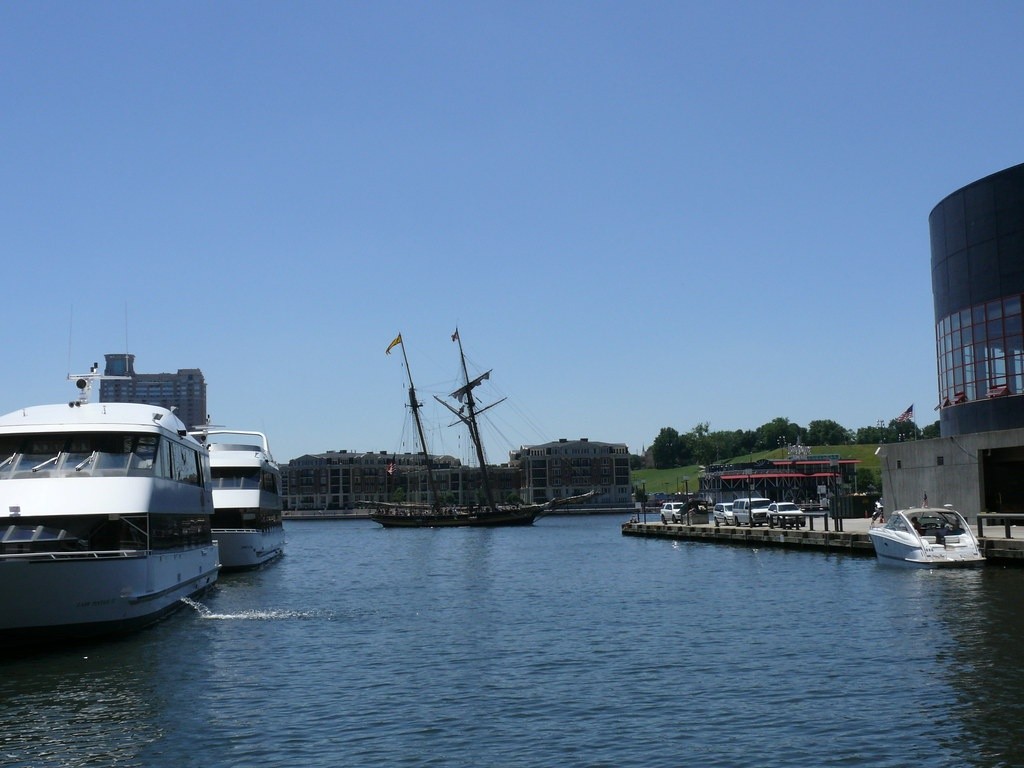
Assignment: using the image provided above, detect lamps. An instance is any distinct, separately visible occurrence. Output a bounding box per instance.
[169,405,179,413]
[177,428,187,440]
[152,413,163,424]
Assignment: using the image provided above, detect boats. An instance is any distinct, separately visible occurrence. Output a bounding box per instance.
[207,429,288,572]
[867,489,987,567]
[0,299,224,648]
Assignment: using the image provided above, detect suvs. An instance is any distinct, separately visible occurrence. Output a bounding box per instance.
[765,500,807,528]
[660,502,686,524]
[712,502,734,527]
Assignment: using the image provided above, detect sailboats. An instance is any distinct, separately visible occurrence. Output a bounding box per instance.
[353,325,608,528]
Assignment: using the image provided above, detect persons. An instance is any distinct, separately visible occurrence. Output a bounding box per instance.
[911,516,922,530]
[785,492,801,504]
[260,516,276,534]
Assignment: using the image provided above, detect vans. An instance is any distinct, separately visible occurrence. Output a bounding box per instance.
[731,497,772,527]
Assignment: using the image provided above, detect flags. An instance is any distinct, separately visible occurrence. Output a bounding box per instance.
[450,331,459,342]
[386,457,397,476]
[385,335,401,355]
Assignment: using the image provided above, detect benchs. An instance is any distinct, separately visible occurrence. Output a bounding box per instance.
[926,527,947,541]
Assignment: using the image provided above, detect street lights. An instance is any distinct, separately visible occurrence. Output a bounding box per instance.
[682,474,691,526]
[876,419,886,444]
[829,456,840,532]
[776,435,786,459]
[745,467,753,528]
[641,479,648,524]
